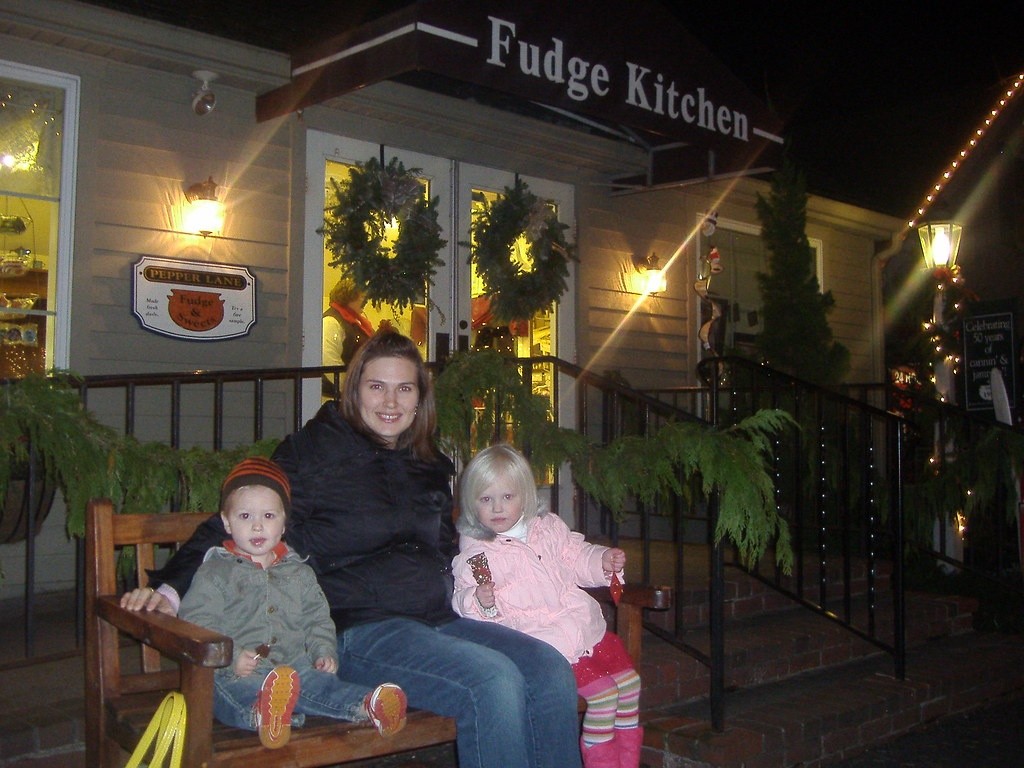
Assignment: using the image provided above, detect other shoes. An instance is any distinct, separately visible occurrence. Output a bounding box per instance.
[365,683,408,739]
[256,667,300,749]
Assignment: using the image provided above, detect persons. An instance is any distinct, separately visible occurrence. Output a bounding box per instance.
[120,329,584,768]
[451,444,643,768]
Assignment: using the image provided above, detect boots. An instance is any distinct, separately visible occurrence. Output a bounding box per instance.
[615,726,643,768]
[580,733,616,768]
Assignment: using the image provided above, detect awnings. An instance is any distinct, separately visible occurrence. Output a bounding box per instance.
[254,4,784,197]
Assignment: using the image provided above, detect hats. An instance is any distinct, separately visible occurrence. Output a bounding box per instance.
[221,457,291,517]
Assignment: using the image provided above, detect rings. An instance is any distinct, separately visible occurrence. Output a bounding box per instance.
[149,587,155,593]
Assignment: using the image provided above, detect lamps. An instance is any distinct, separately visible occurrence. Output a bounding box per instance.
[639,251,667,300]
[184,71,219,116]
[698,179,721,241]
[917,214,967,285]
[182,173,232,240]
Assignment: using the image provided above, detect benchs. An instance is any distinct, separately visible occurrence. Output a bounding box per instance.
[86,498,672,768]
